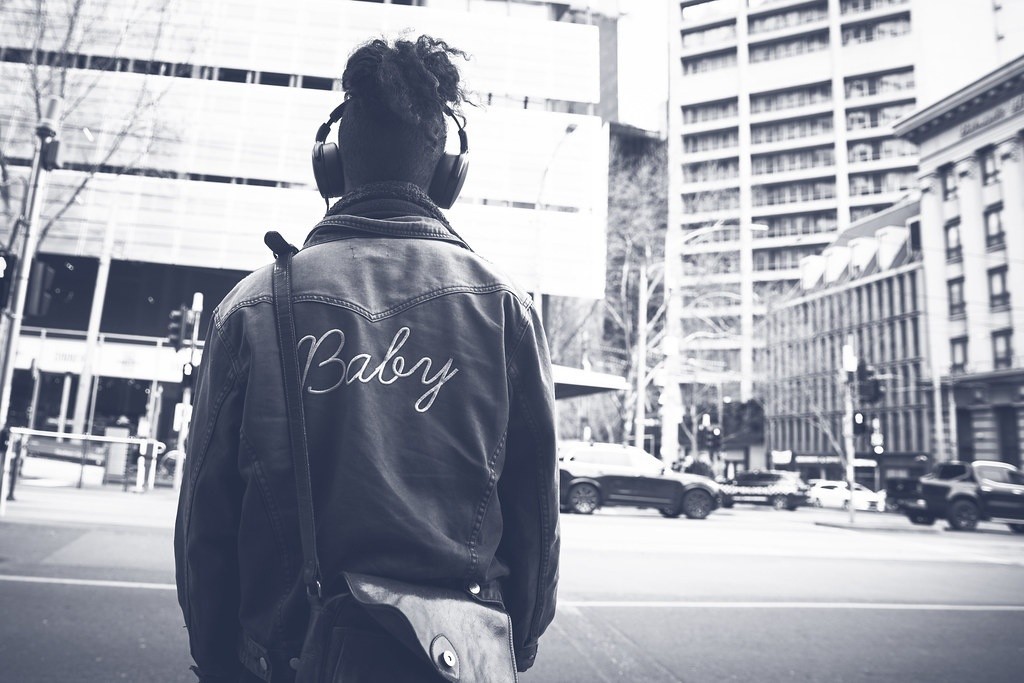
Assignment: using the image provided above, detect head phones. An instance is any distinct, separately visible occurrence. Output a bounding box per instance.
[312,99,468,210]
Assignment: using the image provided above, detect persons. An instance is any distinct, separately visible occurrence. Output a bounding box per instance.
[172,33,560,682]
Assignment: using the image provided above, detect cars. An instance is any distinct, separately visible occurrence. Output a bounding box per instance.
[804,478,886,514]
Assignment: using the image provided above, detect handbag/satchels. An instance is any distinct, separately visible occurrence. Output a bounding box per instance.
[294,570,519,683]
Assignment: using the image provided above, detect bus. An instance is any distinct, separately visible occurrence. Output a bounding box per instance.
[794,456,879,493]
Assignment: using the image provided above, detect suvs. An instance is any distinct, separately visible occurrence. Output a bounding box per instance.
[718,469,811,511]
[557,438,721,520]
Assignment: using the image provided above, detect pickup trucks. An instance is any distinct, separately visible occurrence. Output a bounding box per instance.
[884,458,1024,535]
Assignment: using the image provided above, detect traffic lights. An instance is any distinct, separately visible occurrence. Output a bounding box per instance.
[854,411,866,435]
[857,360,887,404]
[0,253,19,310]
[168,305,188,353]
